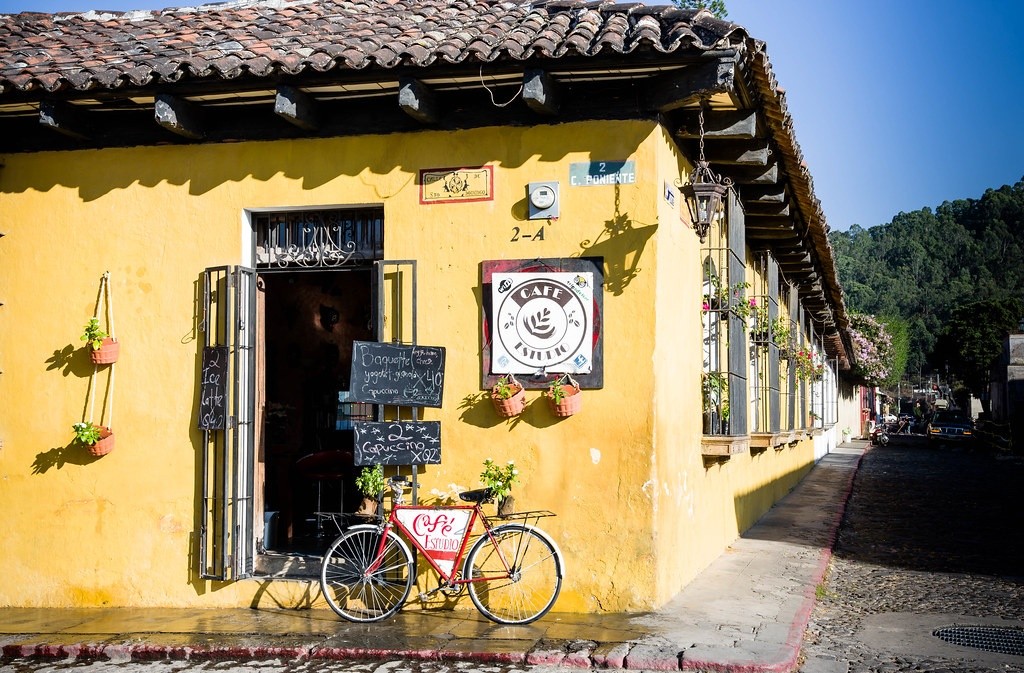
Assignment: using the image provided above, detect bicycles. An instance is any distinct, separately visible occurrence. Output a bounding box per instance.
[319,476,566,626]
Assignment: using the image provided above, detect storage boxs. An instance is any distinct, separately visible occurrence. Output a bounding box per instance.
[264,511,280,549]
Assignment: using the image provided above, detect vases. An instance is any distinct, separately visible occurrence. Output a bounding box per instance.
[84,433,114,456]
[87,337,120,364]
[498,495,515,516]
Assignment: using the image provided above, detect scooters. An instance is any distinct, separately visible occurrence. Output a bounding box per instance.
[873,424,890,446]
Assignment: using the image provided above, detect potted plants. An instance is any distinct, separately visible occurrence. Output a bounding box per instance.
[543,384,583,417]
[355,463,387,515]
[750,305,790,346]
[702,370,730,435]
[706,268,750,309]
[816,369,824,379]
[491,384,527,417]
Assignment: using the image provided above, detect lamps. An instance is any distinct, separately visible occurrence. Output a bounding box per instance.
[679,159,734,243]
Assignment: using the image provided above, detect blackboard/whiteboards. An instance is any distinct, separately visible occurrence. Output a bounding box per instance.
[347,339,447,408]
[197,343,231,433]
[350,416,442,467]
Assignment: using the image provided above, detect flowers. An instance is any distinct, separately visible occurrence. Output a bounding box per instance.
[497,375,513,399]
[72,422,100,449]
[80,318,110,350]
[480,458,518,501]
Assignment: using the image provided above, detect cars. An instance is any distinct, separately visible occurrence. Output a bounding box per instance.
[926,409,979,446]
[883,412,921,434]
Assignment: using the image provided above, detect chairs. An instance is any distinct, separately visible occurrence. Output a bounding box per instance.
[295,451,354,529]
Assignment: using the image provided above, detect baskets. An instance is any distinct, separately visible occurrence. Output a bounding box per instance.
[86,338,120,365]
[489,382,528,419]
[548,384,583,418]
[80,425,114,458]
